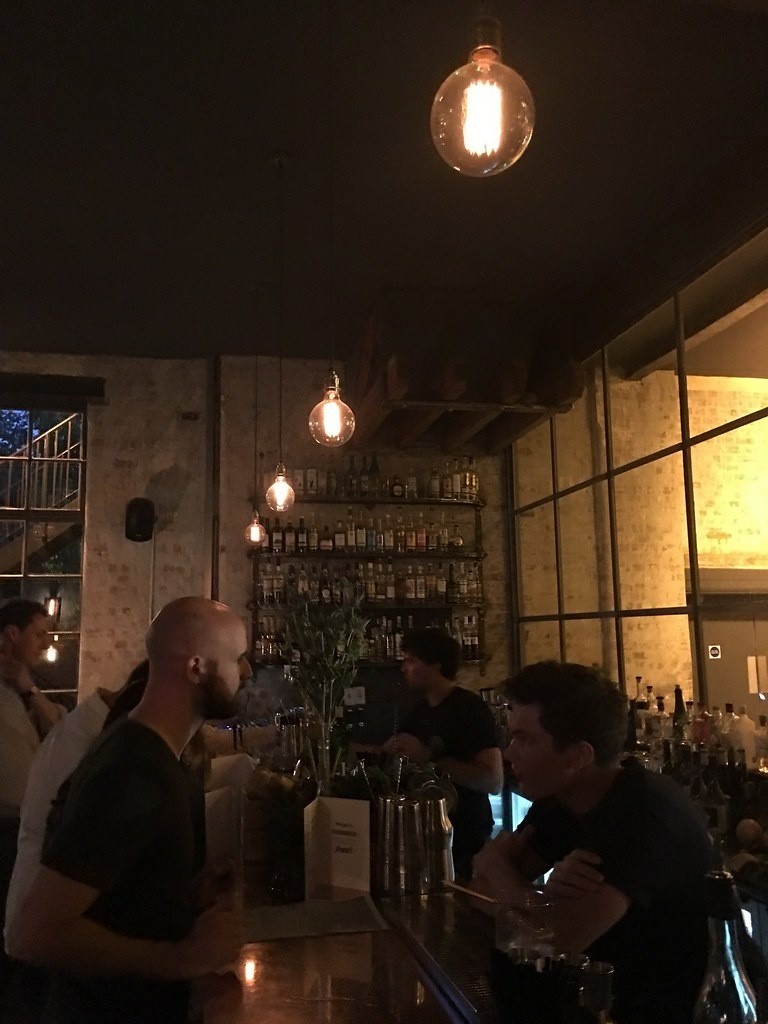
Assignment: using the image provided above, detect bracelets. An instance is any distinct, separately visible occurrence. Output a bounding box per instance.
[429,750,444,763]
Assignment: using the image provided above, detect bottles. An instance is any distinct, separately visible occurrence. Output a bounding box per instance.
[687,870,763,1024]
[257,454,482,666]
[272,713,319,816]
[632,676,768,847]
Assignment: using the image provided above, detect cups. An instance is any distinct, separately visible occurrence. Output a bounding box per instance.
[492,889,556,963]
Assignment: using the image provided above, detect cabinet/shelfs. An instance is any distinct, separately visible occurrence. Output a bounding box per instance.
[247,495,488,676]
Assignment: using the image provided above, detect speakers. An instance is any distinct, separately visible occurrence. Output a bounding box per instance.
[125,497,154,543]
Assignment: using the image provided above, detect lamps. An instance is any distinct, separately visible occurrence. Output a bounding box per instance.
[124,497,156,625]
[308,0,357,448]
[265,158,296,513]
[43,578,62,622]
[429,14,536,177]
[242,291,266,547]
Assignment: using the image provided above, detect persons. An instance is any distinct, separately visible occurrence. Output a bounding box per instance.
[471,660,727,1024]
[0,596,253,1024]
[374,627,504,883]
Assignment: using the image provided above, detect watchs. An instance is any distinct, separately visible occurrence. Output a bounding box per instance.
[24,686,38,700]
